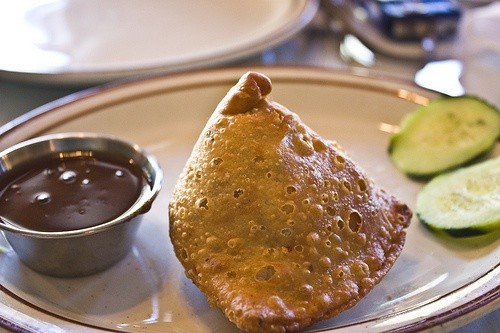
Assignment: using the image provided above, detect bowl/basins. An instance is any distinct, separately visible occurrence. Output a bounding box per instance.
[0,131,162,277]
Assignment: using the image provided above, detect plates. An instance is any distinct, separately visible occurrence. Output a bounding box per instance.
[0,66,500,333]
[1,1,322,87]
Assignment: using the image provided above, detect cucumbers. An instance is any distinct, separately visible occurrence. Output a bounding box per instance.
[414,155,500,239]
[386,94,500,179]
